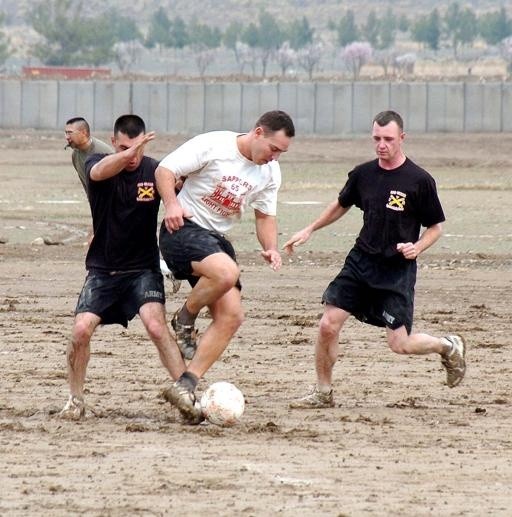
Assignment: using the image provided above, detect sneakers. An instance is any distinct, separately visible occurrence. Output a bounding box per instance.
[441,334,467,388]
[163,379,202,419]
[59,394,86,421]
[289,386,336,410]
[171,307,199,360]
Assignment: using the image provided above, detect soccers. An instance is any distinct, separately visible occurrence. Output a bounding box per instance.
[201,382,244,426]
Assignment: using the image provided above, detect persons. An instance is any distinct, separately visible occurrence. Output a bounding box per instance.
[58,113,207,424]
[153,111,296,425]
[63,118,184,294]
[281,110,467,410]
